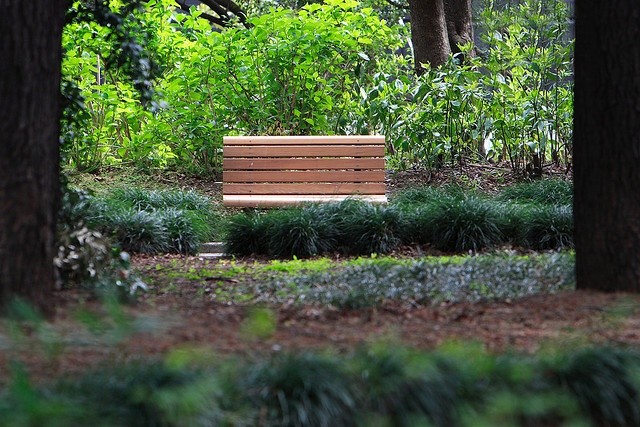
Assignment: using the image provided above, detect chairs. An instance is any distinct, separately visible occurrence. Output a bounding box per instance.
[222,134,388,208]
